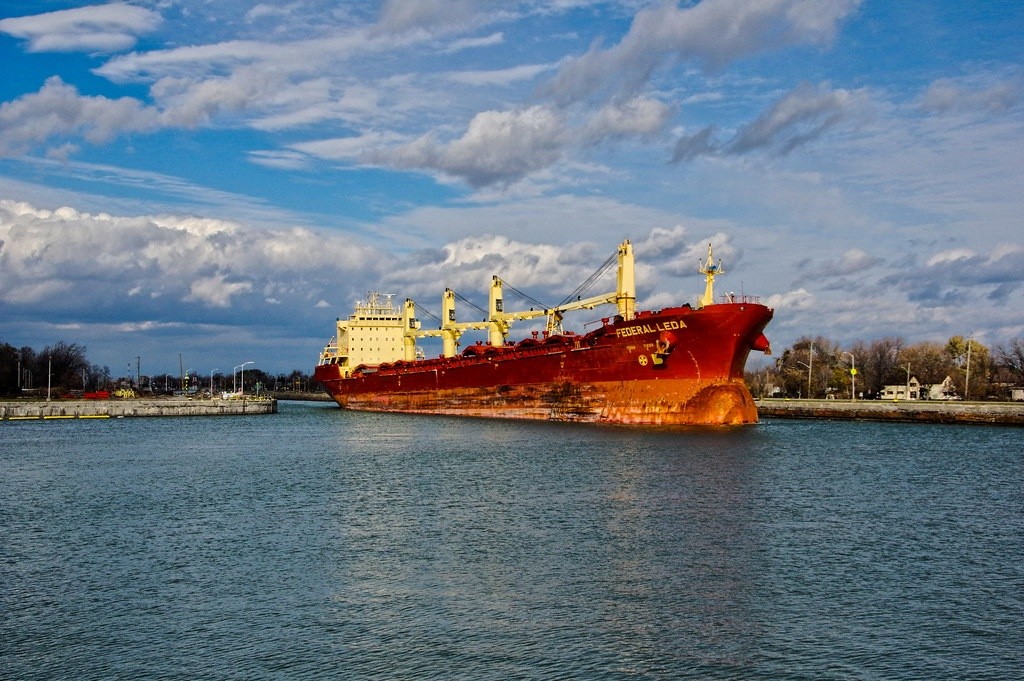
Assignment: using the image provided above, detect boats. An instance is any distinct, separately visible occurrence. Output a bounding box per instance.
[313,251,776,430]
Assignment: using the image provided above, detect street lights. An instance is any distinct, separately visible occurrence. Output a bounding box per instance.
[234,364,241,393]
[241,361,254,396]
[842,351,855,399]
[210,368,218,398]
[901,362,910,397]
